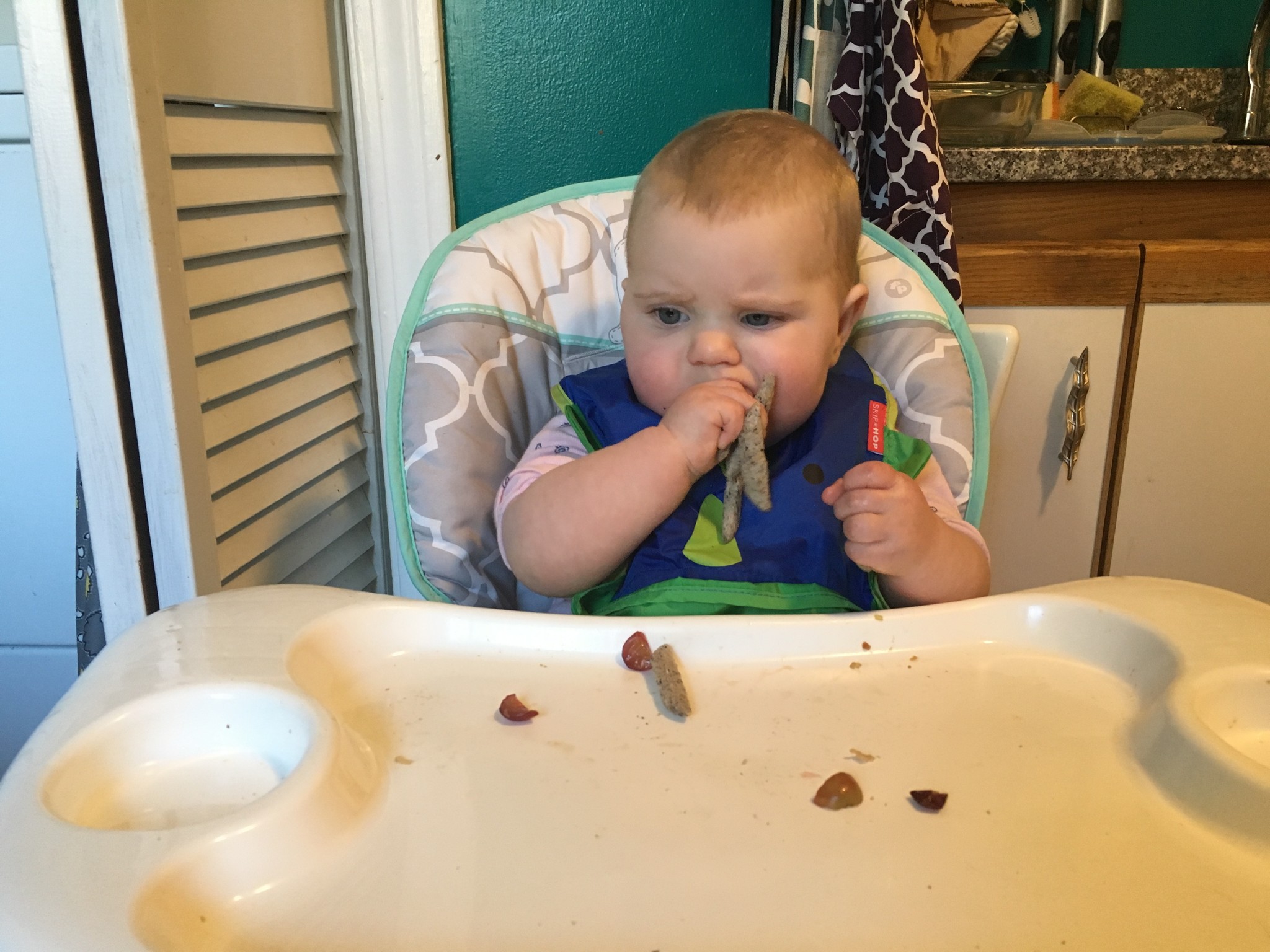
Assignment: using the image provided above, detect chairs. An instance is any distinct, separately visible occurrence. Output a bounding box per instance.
[378,170,988,612]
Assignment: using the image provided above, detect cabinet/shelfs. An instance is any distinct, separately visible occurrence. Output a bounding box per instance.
[948,237,1270,614]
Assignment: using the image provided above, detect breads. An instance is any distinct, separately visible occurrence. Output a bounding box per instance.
[651,644,693,717]
[721,373,775,540]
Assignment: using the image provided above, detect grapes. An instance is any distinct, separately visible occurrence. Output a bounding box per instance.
[621,631,654,671]
[500,694,538,721]
[812,772,864,811]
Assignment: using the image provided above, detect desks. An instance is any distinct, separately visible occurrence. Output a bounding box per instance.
[0,574,1270,952]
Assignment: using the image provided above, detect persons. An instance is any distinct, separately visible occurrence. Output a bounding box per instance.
[493,109,991,616]
